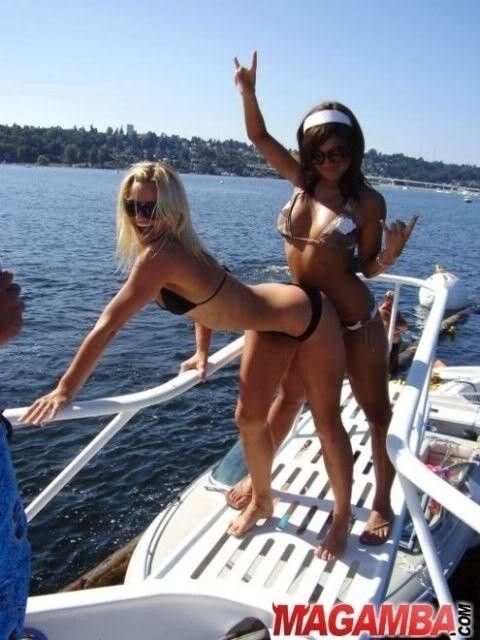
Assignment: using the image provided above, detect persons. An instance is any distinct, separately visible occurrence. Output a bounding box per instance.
[227,48,418,550]
[27,159,350,564]
[373,288,408,376]
[3,263,31,640]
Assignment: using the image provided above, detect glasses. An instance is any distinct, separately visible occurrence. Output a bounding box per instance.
[310,144,350,165]
[124,199,167,220]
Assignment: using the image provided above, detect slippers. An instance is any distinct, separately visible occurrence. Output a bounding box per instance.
[360,510,395,547]
[226,476,254,512]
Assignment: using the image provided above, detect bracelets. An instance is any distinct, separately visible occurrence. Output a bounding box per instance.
[375,252,388,270]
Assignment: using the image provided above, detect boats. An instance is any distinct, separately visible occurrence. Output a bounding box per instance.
[463,196,472,202]
